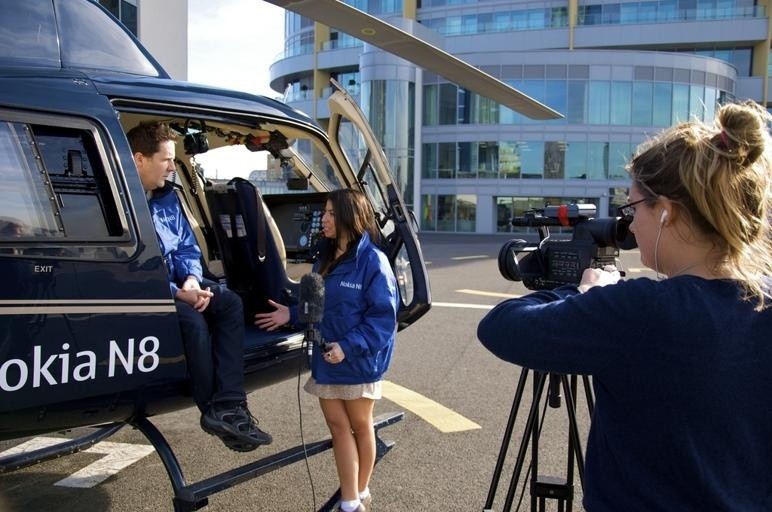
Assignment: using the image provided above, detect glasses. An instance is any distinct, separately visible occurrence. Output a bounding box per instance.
[618,196,654,222]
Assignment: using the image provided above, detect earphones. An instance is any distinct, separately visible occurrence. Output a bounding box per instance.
[660,209,668,224]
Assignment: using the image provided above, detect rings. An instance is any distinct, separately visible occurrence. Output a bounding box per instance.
[329,357,331,360]
[327,352,331,357]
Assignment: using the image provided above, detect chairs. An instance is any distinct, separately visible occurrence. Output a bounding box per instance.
[165,177,315,334]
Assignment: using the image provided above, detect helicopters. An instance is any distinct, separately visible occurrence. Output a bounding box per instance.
[0,1,565,510]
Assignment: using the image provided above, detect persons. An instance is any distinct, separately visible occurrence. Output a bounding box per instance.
[475,100,772,512]
[8,222,25,237]
[254,189,400,512]
[126,119,272,453]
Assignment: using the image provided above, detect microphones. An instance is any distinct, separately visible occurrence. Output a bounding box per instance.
[298,273,325,371]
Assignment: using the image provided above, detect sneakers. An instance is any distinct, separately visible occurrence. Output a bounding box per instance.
[200,406,274,452]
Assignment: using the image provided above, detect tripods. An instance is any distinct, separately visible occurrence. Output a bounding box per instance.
[482,365,594,512]
